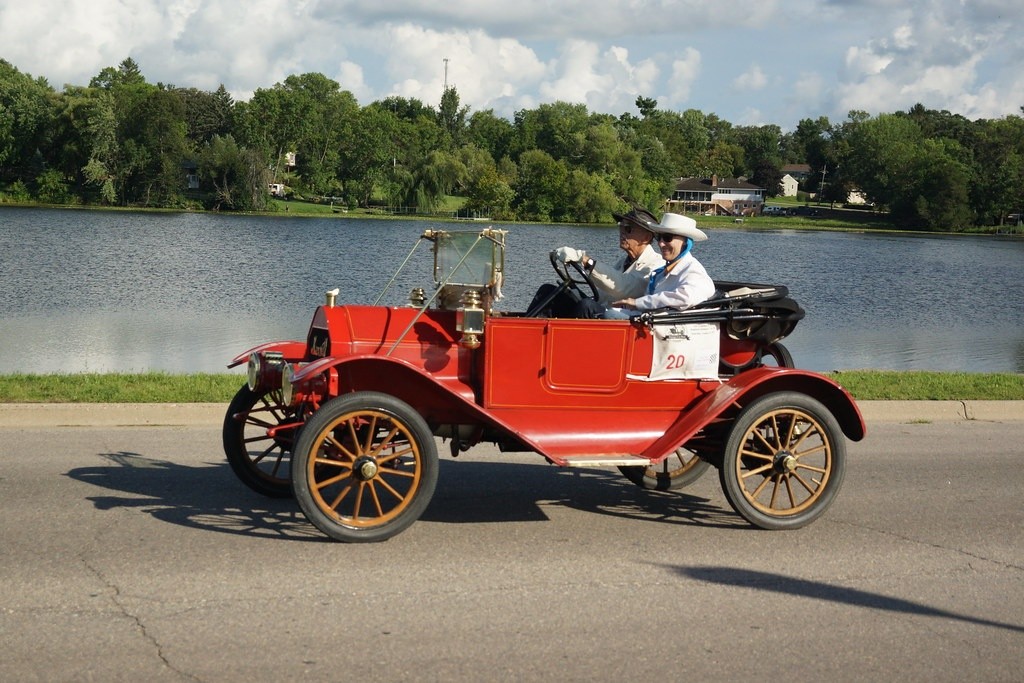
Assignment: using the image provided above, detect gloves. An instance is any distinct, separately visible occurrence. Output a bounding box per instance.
[554,246,585,264]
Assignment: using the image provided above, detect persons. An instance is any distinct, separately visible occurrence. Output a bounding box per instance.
[527,207,716,320]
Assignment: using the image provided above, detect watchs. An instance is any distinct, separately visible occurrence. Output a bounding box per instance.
[584,257,594,270]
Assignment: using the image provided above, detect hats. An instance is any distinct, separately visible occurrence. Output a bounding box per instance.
[611,207,659,233]
[646,212,708,241]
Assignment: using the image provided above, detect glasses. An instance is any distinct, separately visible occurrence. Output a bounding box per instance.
[617,222,641,234]
[654,232,683,242]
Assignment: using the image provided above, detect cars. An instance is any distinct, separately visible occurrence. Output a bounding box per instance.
[782,208,792,214]
[763,206,781,213]
[222,226,866,543]
